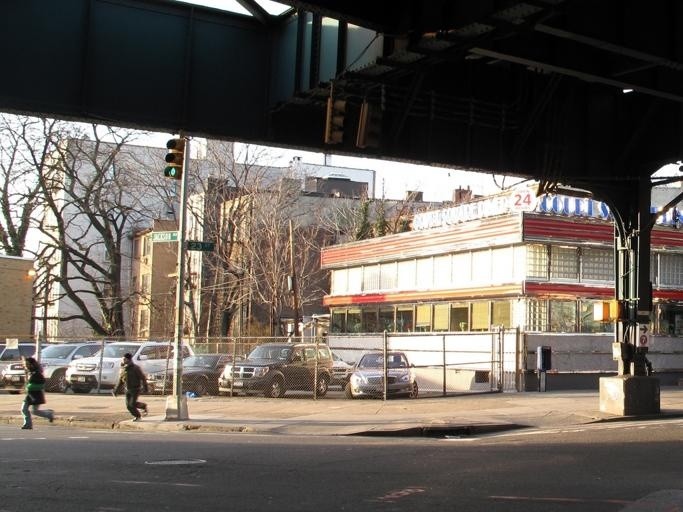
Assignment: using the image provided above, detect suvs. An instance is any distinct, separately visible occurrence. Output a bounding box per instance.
[276,349,353,391]
[1,344,104,393]
[217,341,333,398]
[65,341,193,393]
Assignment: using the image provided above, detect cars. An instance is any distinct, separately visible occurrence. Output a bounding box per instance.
[145,354,245,396]
[344,351,418,399]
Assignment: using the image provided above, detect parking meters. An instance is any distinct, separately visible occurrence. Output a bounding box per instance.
[536,344,553,392]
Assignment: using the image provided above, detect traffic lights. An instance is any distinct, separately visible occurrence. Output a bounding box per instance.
[356,101,384,149]
[162,138,185,182]
[325,96,346,147]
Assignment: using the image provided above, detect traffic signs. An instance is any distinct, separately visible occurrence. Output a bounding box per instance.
[149,231,179,242]
[183,241,214,251]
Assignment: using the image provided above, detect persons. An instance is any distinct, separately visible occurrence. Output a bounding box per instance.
[112,353,148,422]
[20,358,55,429]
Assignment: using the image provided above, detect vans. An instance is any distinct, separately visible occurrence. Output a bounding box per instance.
[0,343,52,387]
